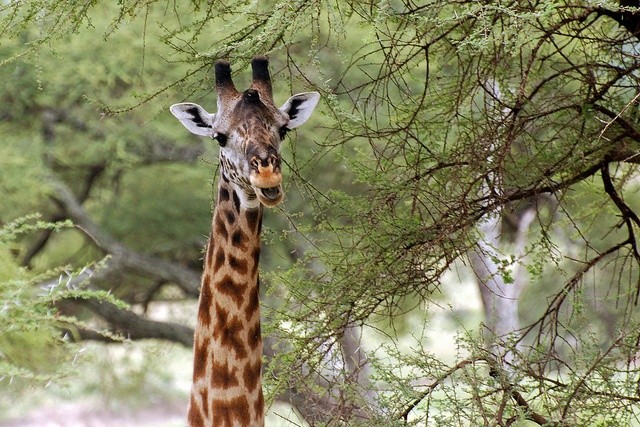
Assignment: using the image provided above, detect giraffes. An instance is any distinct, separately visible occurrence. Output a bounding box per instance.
[170,54,320,427]
